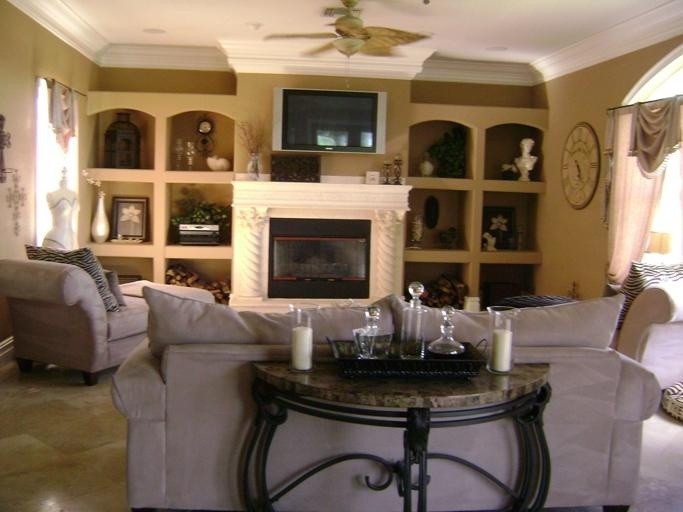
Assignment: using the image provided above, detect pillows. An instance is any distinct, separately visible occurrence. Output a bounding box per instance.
[141,287,393,358]
[603,283,619,297]
[617,262,683,331]
[25,244,118,312]
[660,381,683,421]
[103,268,127,307]
[392,293,625,349]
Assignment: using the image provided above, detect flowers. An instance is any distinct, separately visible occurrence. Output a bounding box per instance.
[82,169,106,198]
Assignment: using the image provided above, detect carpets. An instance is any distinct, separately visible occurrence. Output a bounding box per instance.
[0,357,131,511]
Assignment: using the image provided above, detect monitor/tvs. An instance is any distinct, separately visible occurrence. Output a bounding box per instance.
[272,86,387,157]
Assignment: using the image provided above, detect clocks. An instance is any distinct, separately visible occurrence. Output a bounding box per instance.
[193,113,215,157]
[560,121,600,209]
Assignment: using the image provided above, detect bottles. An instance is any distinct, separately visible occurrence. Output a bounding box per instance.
[399,281,427,360]
[427,305,465,355]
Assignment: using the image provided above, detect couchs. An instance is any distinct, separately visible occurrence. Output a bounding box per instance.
[0,257,215,386]
[507,262,683,392]
[113,285,662,512]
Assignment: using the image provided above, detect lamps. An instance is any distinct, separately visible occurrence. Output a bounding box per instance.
[331,0,366,57]
[645,230,671,255]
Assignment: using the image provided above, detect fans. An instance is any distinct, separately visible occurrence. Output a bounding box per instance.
[261,0,431,59]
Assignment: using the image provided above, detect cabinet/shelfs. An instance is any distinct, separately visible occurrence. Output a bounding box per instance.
[406,104,549,310]
[82,90,234,305]
[231,182,413,313]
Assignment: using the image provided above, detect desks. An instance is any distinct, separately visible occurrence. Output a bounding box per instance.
[239,362,552,511]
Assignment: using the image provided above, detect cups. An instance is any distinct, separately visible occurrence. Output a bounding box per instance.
[486,305,520,374]
[290,303,319,372]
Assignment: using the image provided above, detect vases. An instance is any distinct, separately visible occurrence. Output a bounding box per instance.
[247,153,263,182]
[92,200,110,243]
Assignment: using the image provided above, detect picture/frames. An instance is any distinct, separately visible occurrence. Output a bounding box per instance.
[112,196,148,239]
[482,206,516,249]
